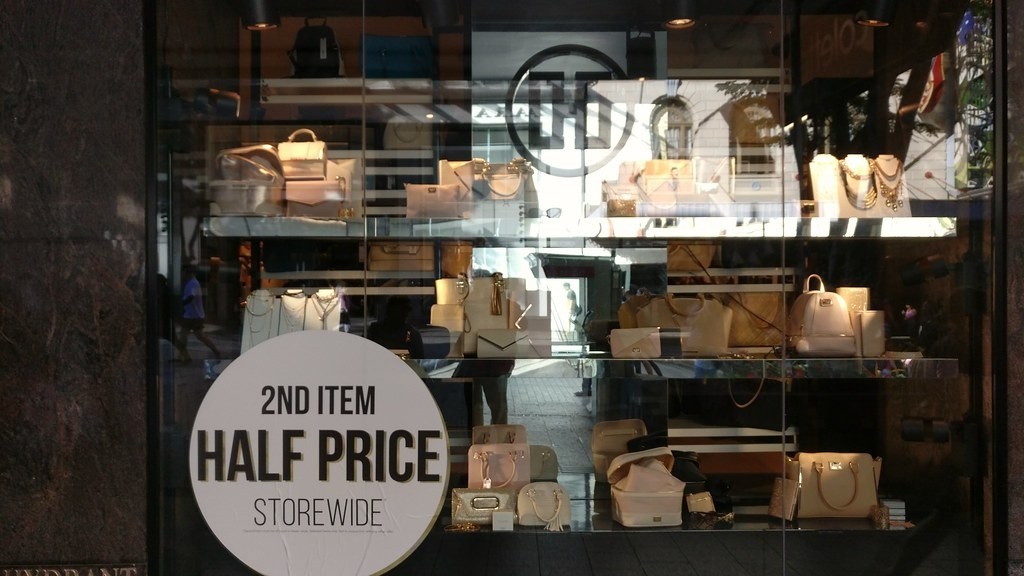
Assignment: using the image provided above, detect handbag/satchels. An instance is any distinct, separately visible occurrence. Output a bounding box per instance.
[600,155,736,217]
[529,444,558,481]
[429,272,552,359]
[467,444,529,489]
[469,424,527,446]
[616,287,734,360]
[784,450,880,520]
[277,126,328,181]
[405,182,460,220]
[452,487,517,525]
[728,171,788,202]
[439,156,534,217]
[518,481,572,531]
[727,285,787,348]
[283,178,347,221]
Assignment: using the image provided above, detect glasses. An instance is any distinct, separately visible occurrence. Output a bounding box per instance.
[701,512,734,530]
[686,509,718,529]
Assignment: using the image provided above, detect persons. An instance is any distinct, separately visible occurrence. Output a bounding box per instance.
[463,269,527,427]
[156,265,225,365]
[563,282,579,336]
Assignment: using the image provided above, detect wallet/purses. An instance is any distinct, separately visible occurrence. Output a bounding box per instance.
[608,326,662,358]
[686,491,716,512]
[852,311,886,356]
[838,285,870,331]
[768,476,801,520]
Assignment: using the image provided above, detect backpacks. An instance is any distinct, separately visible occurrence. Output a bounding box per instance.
[787,272,855,356]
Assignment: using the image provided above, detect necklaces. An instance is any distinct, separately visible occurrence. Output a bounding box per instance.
[244,289,340,348]
[838,158,906,213]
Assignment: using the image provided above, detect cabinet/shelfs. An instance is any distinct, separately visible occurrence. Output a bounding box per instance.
[204,68,958,533]
